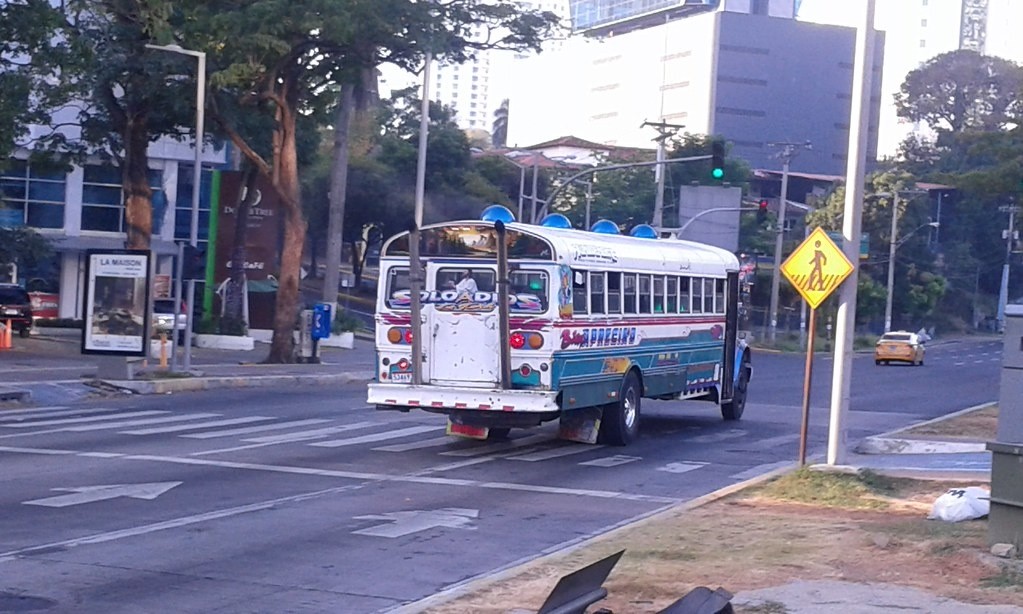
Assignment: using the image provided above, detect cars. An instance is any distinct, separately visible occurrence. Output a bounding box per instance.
[873,330,927,367]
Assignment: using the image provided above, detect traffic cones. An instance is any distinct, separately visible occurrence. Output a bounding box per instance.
[4,319,16,349]
[0,324,6,349]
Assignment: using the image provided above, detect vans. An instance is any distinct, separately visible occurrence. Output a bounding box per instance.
[27,292,58,320]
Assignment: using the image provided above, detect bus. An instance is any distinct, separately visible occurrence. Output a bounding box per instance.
[366,205,753,448]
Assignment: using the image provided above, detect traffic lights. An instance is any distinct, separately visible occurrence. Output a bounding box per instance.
[182,241,207,281]
[711,139,726,180]
[756,199,769,225]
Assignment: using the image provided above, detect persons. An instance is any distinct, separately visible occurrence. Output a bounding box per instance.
[449,269,478,294]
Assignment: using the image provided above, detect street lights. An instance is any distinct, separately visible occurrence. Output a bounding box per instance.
[144,41,206,373]
[793,190,893,348]
[992,239,1023,335]
[469,147,577,224]
[882,221,941,335]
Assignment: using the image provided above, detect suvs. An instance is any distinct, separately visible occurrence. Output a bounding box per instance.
[153,297,188,344]
[0,283,34,337]
